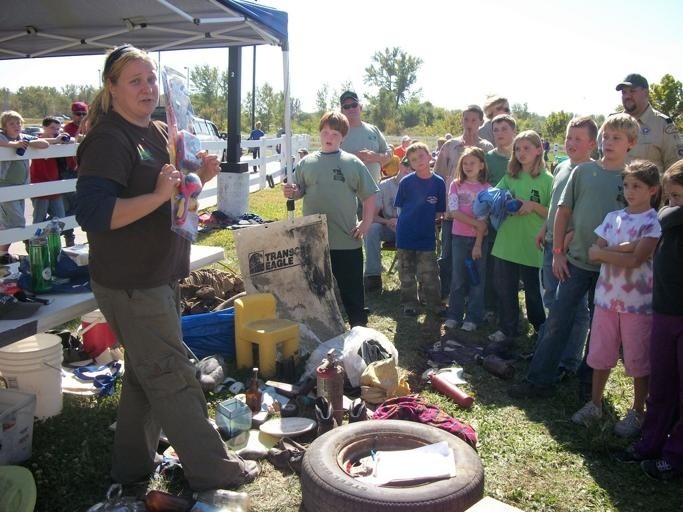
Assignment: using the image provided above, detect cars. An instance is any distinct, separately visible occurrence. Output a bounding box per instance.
[20,115,70,136]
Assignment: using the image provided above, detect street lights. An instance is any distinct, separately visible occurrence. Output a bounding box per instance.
[183,67,190,90]
[96,69,101,90]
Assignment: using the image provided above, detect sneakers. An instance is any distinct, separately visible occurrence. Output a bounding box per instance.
[614,410,649,436]
[0,254,19,264]
[612,443,650,466]
[519,348,576,383]
[571,400,604,425]
[487,328,518,344]
[461,321,480,332]
[225,458,263,488]
[509,378,555,399]
[442,317,460,329]
[639,458,681,484]
[404,294,495,321]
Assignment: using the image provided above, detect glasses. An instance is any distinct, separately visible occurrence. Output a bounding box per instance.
[342,102,357,109]
[73,112,88,115]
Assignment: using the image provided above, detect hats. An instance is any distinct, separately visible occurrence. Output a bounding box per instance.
[339,91,358,101]
[615,74,648,94]
[70,101,87,112]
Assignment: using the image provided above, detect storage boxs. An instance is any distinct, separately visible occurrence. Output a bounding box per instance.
[0,386,36,469]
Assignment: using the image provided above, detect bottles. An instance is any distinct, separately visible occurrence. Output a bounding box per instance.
[144,489,248,512]
[465,258,481,286]
[245,367,262,412]
[54,133,69,142]
[44,222,61,269]
[427,371,474,409]
[15,137,30,156]
[28,234,53,293]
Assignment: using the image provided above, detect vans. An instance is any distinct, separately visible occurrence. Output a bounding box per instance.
[191,116,227,162]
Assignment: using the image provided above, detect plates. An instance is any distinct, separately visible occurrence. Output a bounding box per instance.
[259,417,315,437]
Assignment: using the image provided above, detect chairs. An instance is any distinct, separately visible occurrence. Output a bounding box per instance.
[382,217,442,283]
[234,292,300,379]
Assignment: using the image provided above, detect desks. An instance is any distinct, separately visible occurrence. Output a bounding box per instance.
[0,236,228,354]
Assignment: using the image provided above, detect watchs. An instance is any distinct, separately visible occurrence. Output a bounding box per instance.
[552,247,564,254]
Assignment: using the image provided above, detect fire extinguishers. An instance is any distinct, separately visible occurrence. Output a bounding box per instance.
[315,348,344,427]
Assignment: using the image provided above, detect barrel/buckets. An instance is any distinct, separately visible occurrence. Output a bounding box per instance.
[76,308,118,357]
[0,332,64,426]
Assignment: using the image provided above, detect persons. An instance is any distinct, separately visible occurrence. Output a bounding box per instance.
[0,102,88,264]
[297,148,308,158]
[596,73,682,173]
[278,121,293,153]
[364,93,553,360]
[284,154,296,176]
[340,90,392,221]
[74,45,261,493]
[280,111,380,330]
[246,121,264,172]
[508,112,682,481]
